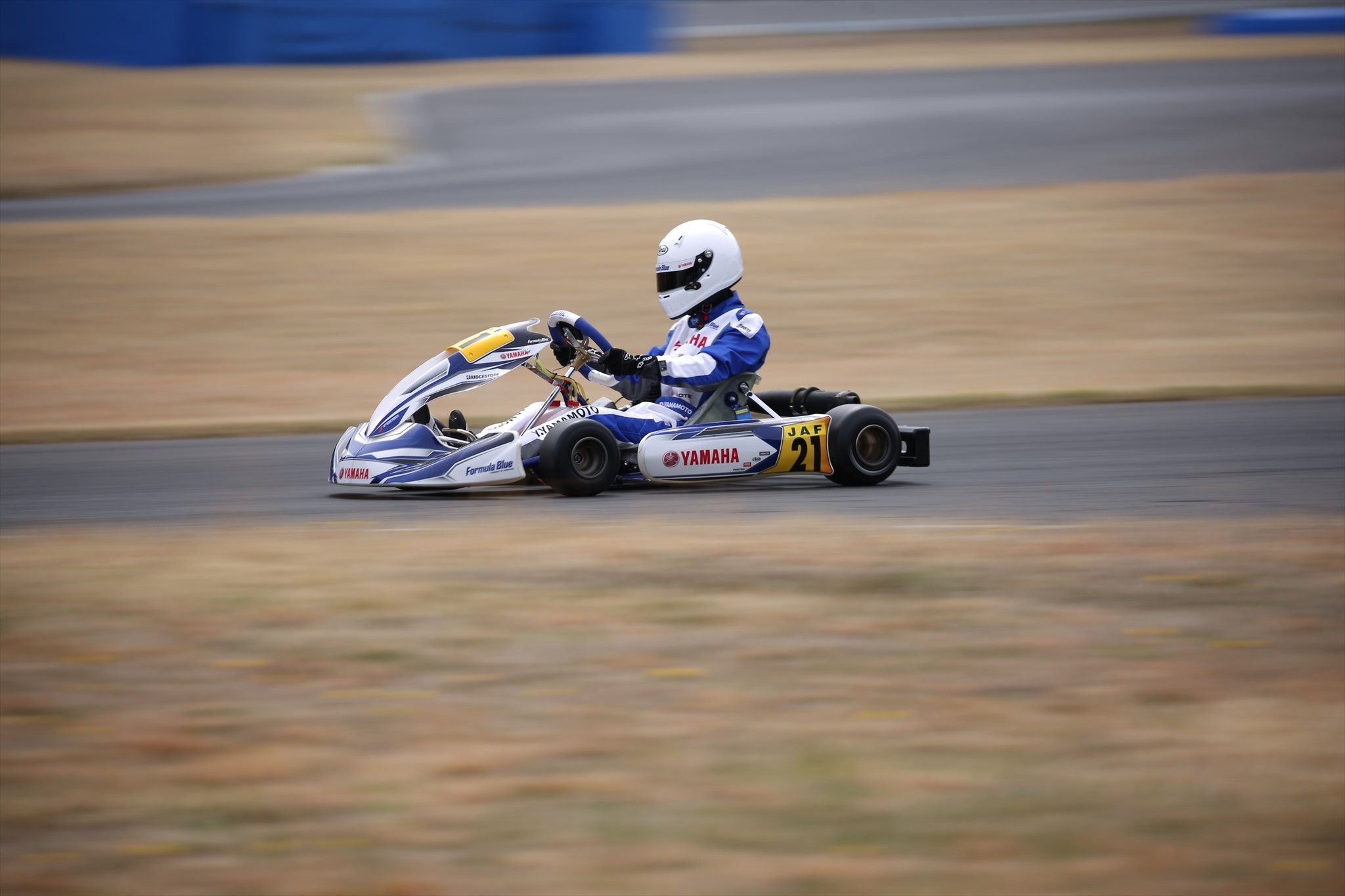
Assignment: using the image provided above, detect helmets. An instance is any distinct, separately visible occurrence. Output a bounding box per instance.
[655,219,744,321]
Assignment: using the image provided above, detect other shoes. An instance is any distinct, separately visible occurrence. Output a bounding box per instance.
[448,408,476,446]
[410,403,447,444]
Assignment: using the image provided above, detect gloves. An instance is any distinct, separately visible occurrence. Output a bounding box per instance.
[598,348,662,380]
[550,338,576,367]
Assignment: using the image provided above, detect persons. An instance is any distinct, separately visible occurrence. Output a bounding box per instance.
[411,217,771,448]
